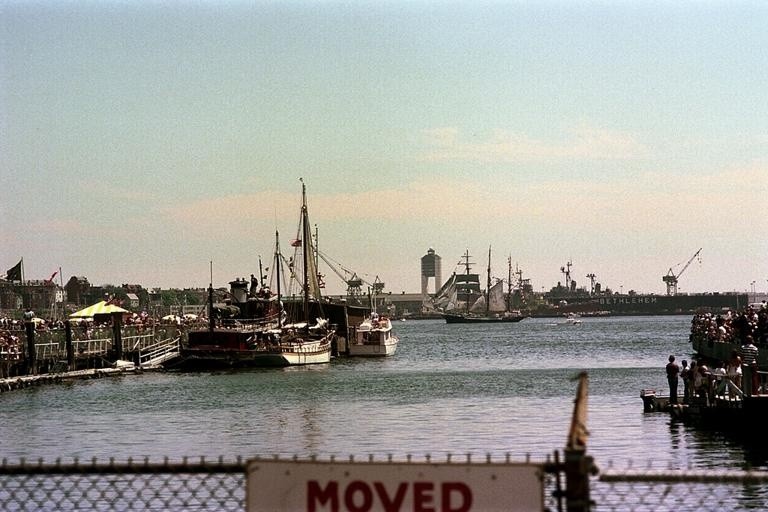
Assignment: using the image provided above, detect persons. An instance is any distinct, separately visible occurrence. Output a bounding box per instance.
[249,274,258,297]
[0,306,208,361]
[664,305,768,404]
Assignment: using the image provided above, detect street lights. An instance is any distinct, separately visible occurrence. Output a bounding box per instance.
[748,282,752,294]
[752,280,756,303]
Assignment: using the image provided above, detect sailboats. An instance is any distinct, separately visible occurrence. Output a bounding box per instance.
[416,243,613,326]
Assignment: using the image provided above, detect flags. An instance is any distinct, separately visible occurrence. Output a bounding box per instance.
[5,260,23,282]
[47,270,60,282]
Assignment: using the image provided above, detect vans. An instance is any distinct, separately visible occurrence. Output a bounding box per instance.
[748,302,762,310]
